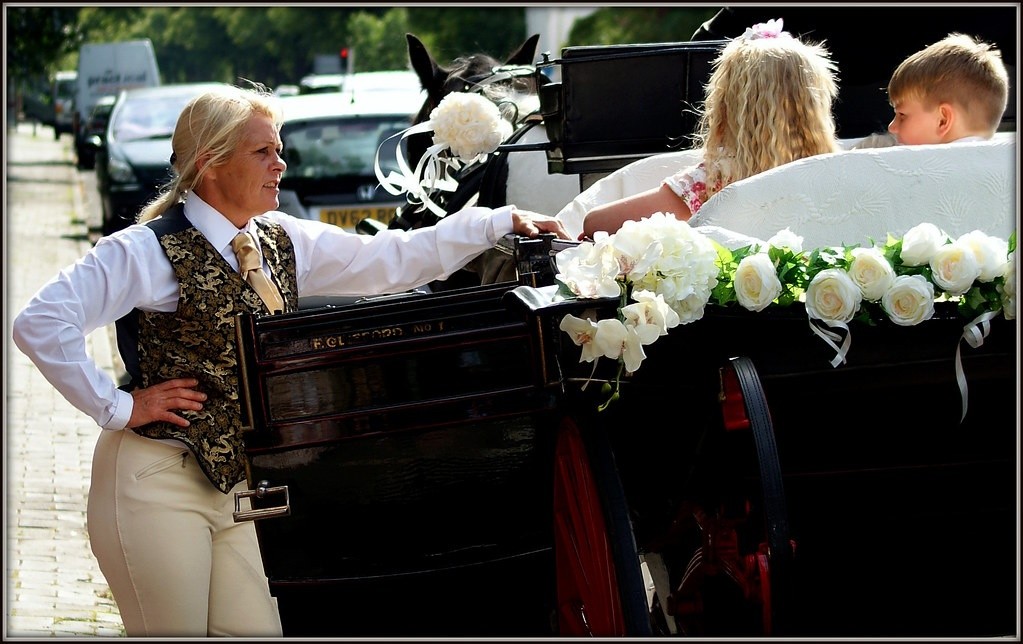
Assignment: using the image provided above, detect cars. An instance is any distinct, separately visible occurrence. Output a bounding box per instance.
[306,72,427,177]
[271,91,429,234]
[282,75,343,167]
[82,93,117,169]
[86,82,232,238]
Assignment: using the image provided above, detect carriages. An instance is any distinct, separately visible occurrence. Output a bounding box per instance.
[232,6,1016,638]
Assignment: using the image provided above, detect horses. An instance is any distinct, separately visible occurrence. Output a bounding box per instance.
[406,32,552,192]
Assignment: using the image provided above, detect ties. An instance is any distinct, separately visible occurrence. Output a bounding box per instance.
[231,231,285,315]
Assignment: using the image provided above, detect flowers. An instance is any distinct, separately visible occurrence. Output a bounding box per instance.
[551,216,1015,413]
[430,90,514,163]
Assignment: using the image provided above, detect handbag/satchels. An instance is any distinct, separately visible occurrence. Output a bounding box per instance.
[673,355,805,637]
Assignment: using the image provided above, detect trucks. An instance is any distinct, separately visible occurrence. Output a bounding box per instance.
[74,39,161,152]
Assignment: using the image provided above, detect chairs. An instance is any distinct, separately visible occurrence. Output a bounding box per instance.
[377,127,406,169]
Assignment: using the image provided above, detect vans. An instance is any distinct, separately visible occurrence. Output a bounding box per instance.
[51,71,76,139]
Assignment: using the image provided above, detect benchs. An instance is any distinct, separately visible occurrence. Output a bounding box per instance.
[682,127,1016,257]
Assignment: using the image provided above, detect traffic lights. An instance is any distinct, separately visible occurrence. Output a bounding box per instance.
[340,48,347,71]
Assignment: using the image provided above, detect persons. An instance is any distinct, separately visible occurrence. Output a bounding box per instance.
[14,90,571,637]
[888,32,1008,146]
[583,18,843,240]
